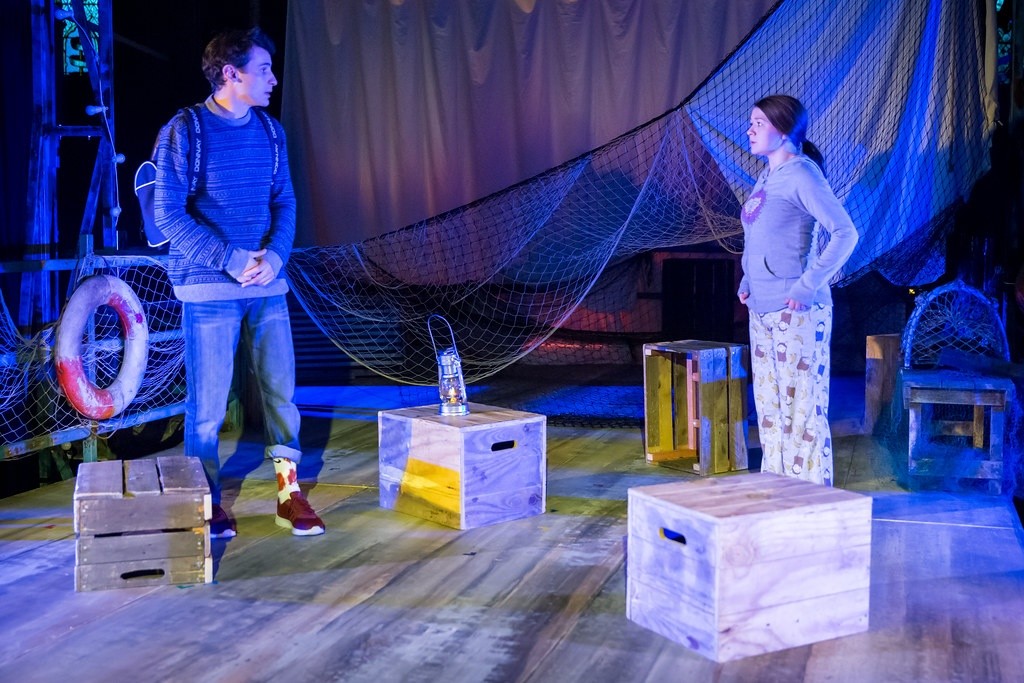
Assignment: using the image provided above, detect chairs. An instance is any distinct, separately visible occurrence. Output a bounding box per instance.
[898,280,1008,496]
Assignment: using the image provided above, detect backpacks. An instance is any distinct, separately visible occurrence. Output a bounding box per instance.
[134,107,282,248]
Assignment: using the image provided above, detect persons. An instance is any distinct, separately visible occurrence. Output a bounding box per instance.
[737,94,859,488]
[154,24,324,538]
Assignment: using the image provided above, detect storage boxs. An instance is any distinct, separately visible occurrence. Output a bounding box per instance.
[640,337,752,475]
[74,456,215,596]
[378,400,548,531]
[621,469,872,662]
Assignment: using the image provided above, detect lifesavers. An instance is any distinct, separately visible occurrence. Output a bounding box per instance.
[52,275,149,422]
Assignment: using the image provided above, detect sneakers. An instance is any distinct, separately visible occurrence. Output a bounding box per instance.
[210,504,237,538]
[275,490,325,536]
